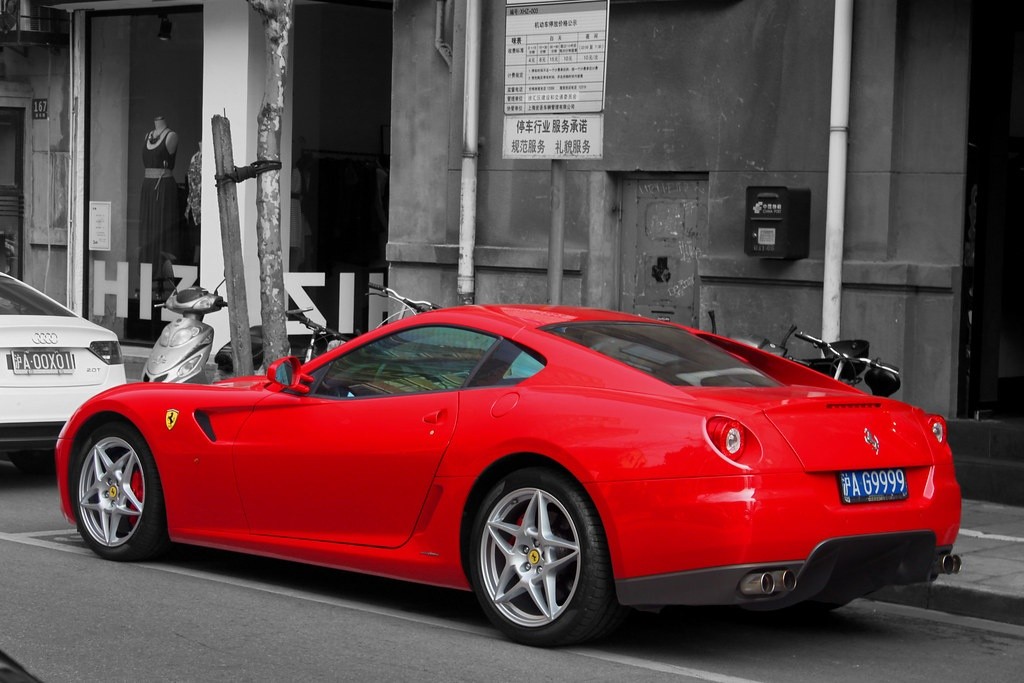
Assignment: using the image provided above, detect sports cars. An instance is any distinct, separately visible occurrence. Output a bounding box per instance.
[53,302,963,649]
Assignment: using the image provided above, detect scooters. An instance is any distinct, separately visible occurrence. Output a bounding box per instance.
[138,268,295,383]
[705,310,902,398]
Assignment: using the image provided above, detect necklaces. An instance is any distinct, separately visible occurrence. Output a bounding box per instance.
[149,126,168,145]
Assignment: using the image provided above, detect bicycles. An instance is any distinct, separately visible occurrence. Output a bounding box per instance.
[364,281,444,328]
[284,306,355,365]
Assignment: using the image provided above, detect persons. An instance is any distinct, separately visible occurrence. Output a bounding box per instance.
[139,116,188,281]
[184,141,202,265]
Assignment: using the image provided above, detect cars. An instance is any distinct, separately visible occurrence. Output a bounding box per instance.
[1,271,127,473]
